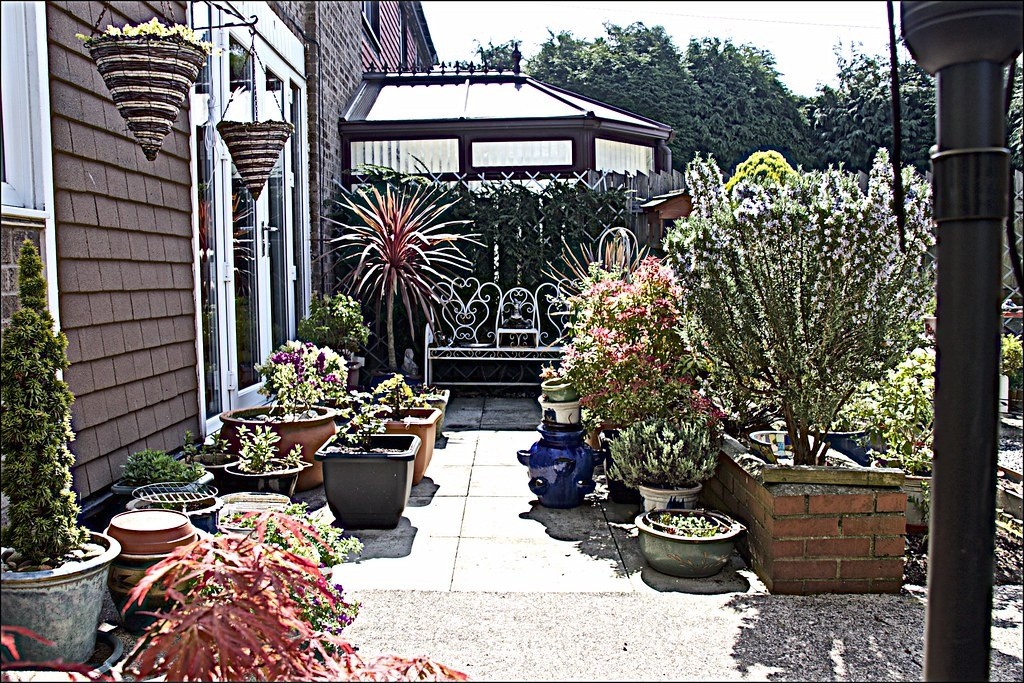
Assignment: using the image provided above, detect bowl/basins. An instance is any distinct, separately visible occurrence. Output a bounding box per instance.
[107,508,199,556]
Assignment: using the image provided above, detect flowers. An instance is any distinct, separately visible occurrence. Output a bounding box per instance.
[76,18,223,57]
[257,341,345,422]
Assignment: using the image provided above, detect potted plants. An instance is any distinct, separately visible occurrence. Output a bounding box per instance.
[0,177,488,680]
[517,242,1023,580]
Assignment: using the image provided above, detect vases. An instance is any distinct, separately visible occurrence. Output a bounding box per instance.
[219,407,339,491]
[104,508,211,635]
[217,120,295,201]
[85,33,207,161]
